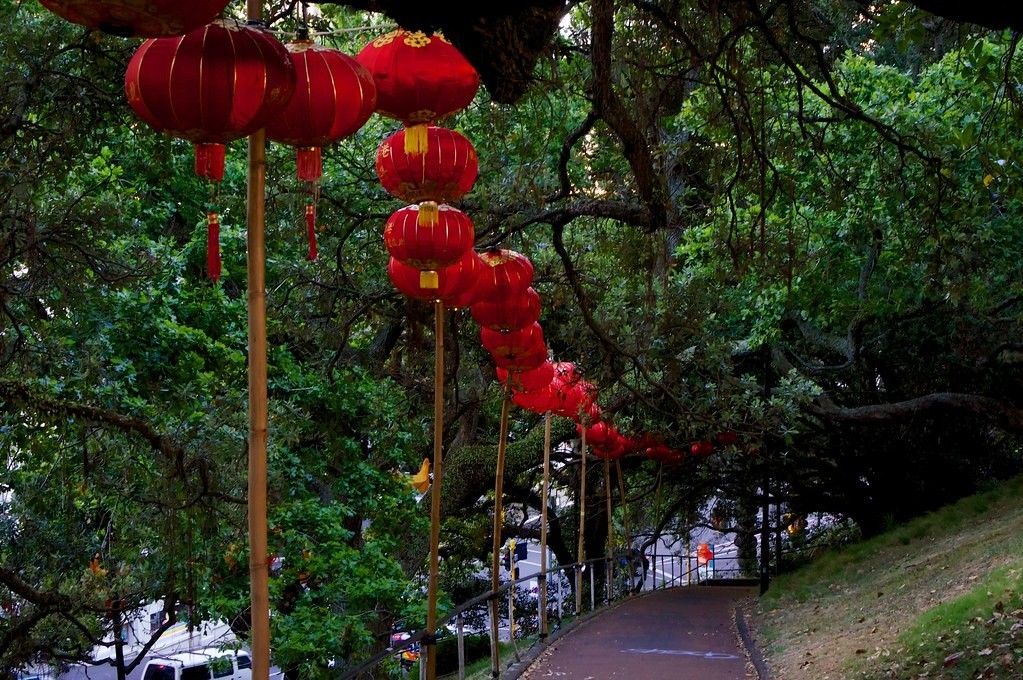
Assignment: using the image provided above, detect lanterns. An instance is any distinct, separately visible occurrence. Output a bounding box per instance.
[352,27,481,153]
[480,322,543,359]
[496,359,555,393]
[386,248,481,305]
[470,286,541,335]
[476,247,534,304]
[492,341,548,373]
[376,119,479,229]
[37,0,233,39]
[509,361,739,462]
[265,0,376,259]
[384,202,474,294]
[124,21,295,282]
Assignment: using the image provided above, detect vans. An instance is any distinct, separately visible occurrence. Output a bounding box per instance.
[140,649,254,680]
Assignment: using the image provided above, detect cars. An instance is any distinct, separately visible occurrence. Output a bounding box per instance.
[391,624,473,671]
[612,541,649,575]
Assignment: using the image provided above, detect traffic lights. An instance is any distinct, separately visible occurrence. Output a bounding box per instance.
[503,547,511,571]
